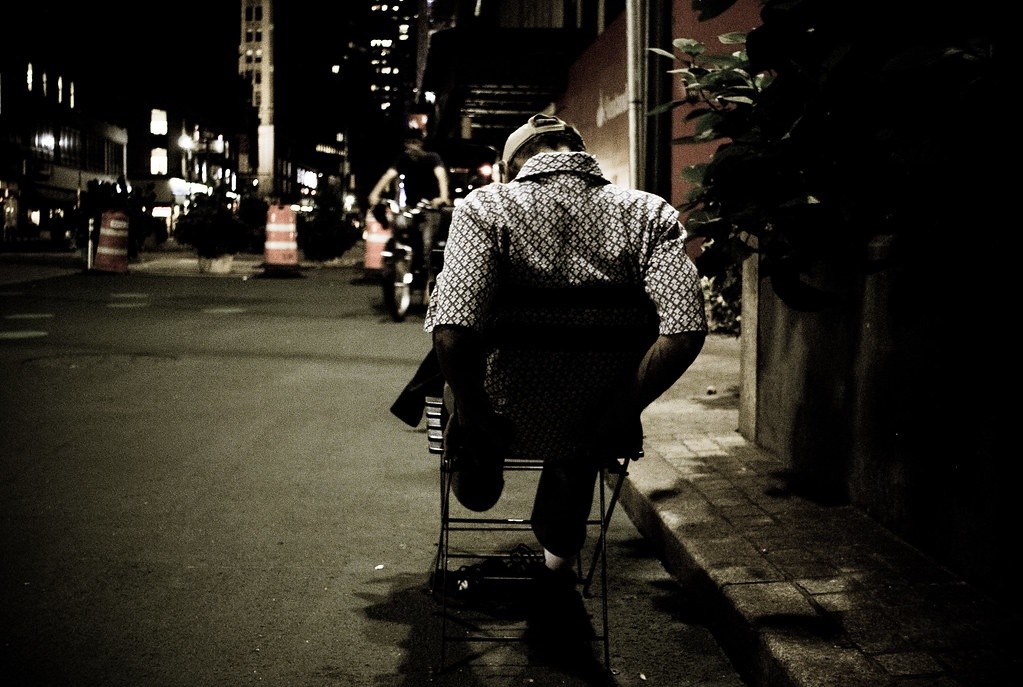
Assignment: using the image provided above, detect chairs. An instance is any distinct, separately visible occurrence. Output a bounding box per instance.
[422,225,660,687]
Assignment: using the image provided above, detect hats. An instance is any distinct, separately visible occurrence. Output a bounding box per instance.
[502,112,586,181]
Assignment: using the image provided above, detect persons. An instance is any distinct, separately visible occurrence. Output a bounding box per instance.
[368,127,448,283]
[432,111,708,612]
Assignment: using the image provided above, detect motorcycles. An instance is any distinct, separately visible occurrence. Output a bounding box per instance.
[372,194,448,322]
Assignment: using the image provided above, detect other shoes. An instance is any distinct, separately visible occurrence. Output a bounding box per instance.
[431,540,572,616]
